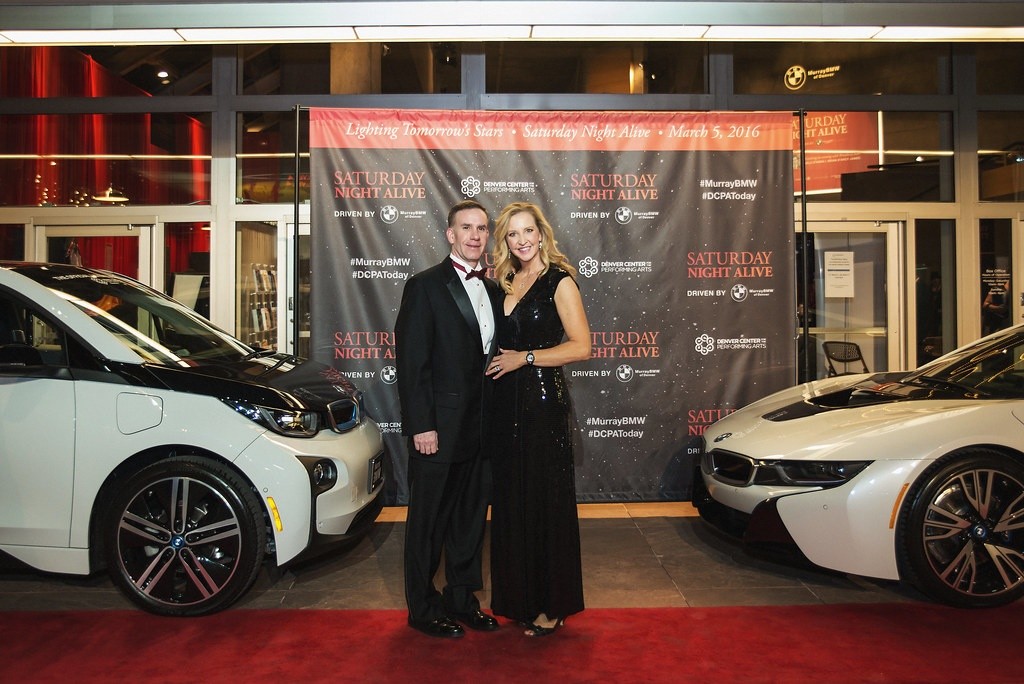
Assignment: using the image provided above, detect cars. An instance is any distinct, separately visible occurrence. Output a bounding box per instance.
[1,256,389,621]
[690,325,1020,611]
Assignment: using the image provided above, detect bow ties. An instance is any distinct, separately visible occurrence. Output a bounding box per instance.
[451,259,488,281]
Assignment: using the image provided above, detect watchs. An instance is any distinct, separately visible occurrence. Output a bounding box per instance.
[526,350,535,364]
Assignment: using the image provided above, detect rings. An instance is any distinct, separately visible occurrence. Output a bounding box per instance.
[493,365,500,372]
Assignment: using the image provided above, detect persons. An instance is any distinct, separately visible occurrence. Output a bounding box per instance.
[984,279,1008,331]
[394,201,505,636]
[487,202,592,637]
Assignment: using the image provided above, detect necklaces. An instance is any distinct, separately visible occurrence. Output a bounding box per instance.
[519,269,533,289]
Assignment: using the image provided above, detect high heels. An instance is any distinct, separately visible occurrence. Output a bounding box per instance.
[524,614,564,637]
[519,615,539,627]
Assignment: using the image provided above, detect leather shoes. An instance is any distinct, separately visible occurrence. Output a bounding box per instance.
[408,614,465,637]
[457,609,500,631]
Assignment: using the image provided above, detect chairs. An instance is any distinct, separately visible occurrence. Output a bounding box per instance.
[823,341,871,377]
[0,342,43,366]
